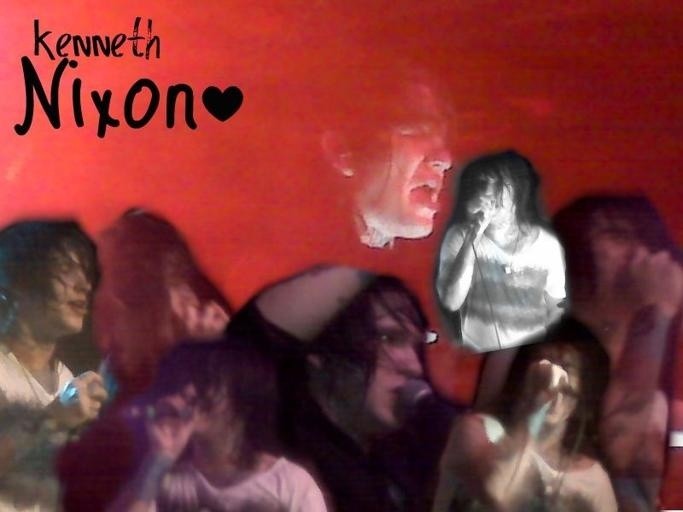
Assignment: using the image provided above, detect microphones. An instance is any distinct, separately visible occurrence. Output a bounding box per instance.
[118,392,219,420]
[400,380,460,425]
[470,202,490,236]
[556,377,585,404]
[68,335,102,439]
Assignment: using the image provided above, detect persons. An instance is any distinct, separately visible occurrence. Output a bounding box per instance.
[0,190,683,512]
[319,81,452,249]
[434,150,574,353]
